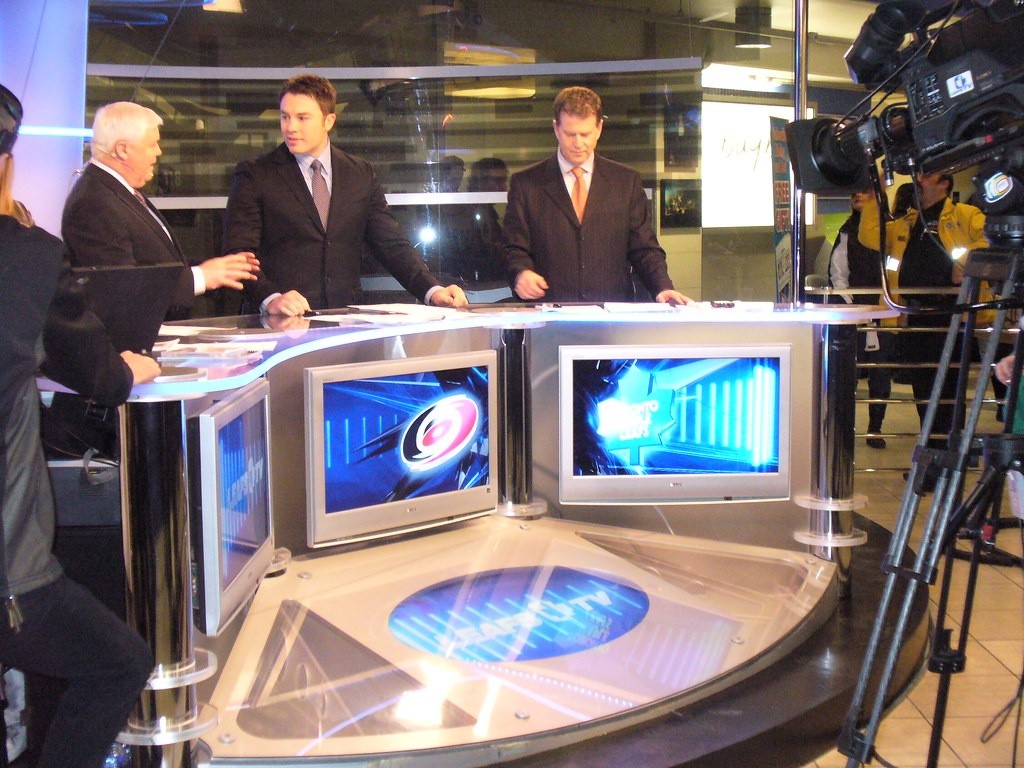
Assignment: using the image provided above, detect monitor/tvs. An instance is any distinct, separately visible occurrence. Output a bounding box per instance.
[559,342,791,506]
[187,378,276,638]
[303,348,497,549]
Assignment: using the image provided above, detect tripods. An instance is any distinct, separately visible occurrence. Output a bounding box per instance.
[835,215,1024,768]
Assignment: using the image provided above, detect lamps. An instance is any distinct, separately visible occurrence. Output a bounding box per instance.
[734,6,772,48]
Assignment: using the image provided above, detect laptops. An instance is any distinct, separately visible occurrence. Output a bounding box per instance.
[73,260,183,355]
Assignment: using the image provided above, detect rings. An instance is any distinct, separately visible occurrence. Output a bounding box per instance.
[1006,380,1011,384]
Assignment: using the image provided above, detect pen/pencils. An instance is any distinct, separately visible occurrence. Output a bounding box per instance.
[525,302,562,308]
[309,310,320,314]
[358,309,408,315]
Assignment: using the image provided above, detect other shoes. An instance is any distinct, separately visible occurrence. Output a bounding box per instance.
[866,437,885,449]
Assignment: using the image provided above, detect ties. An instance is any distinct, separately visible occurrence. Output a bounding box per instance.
[311,160,331,233]
[571,167,588,226]
[134,190,149,212]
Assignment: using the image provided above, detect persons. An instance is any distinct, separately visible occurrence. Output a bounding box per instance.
[829,170,1024,449]
[443,156,507,278]
[0,81,162,768]
[61,102,261,324]
[222,72,471,317]
[500,87,695,305]
